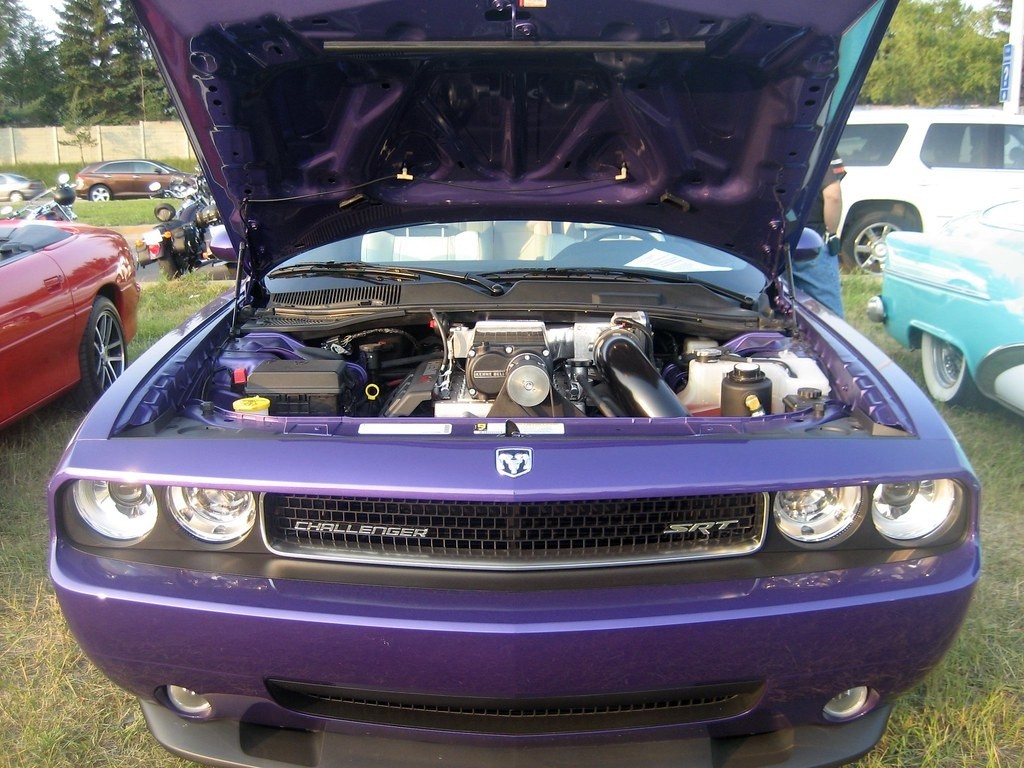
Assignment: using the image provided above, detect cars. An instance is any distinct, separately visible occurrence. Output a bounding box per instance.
[864,190,1024,421]
[0,171,47,203]
[38,0,986,767]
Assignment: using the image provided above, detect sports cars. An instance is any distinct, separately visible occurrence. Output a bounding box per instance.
[0,218,144,435]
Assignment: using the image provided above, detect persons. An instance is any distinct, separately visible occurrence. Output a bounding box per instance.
[783,151,846,319]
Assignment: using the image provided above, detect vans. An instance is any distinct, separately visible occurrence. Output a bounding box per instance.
[835,106,1024,276]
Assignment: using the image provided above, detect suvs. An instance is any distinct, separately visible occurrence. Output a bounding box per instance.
[75,159,197,202]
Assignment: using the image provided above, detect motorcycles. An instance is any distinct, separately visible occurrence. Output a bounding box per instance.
[133,164,220,281]
[0,171,80,222]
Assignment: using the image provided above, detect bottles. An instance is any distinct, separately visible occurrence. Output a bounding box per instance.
[721,363,771,417]
